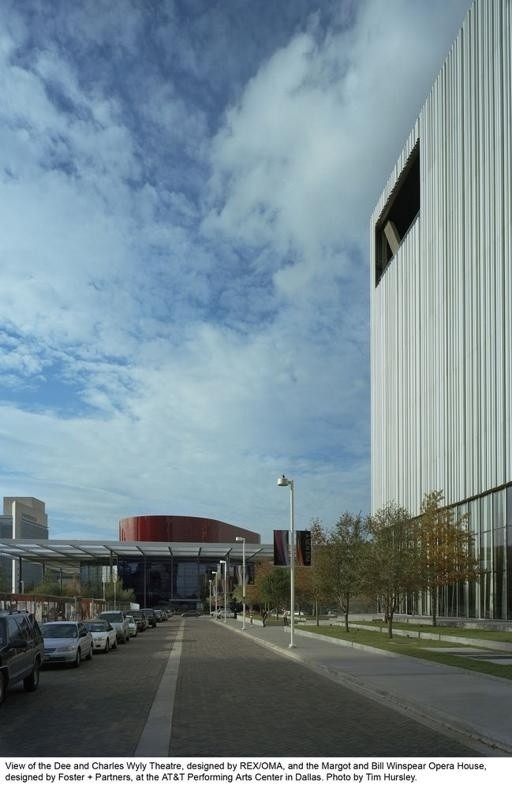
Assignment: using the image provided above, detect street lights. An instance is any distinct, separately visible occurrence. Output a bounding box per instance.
[274,474,297,648]
[235,536,248,631]
[211,571,218,621]
[208,579,213,615]
[219,559,227,623]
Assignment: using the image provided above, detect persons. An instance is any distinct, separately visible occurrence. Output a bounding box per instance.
[262,610,267,627]
[283,609,289,626]
[56,610,66,622]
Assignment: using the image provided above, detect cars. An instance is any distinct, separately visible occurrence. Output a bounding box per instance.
[153,609,174,623]
[181,609,201,618]
[125,615,137,637]
[267,608,310,616]
[208,608,236,618]
[38,620,93,669]
[82,620,118,653]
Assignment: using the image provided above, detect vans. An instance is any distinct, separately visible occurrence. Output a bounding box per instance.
[97,610,128,644]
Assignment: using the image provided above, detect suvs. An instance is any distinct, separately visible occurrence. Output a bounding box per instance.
[0,608,46,703]
[127,610,149,632]
[141,608,157,628]
